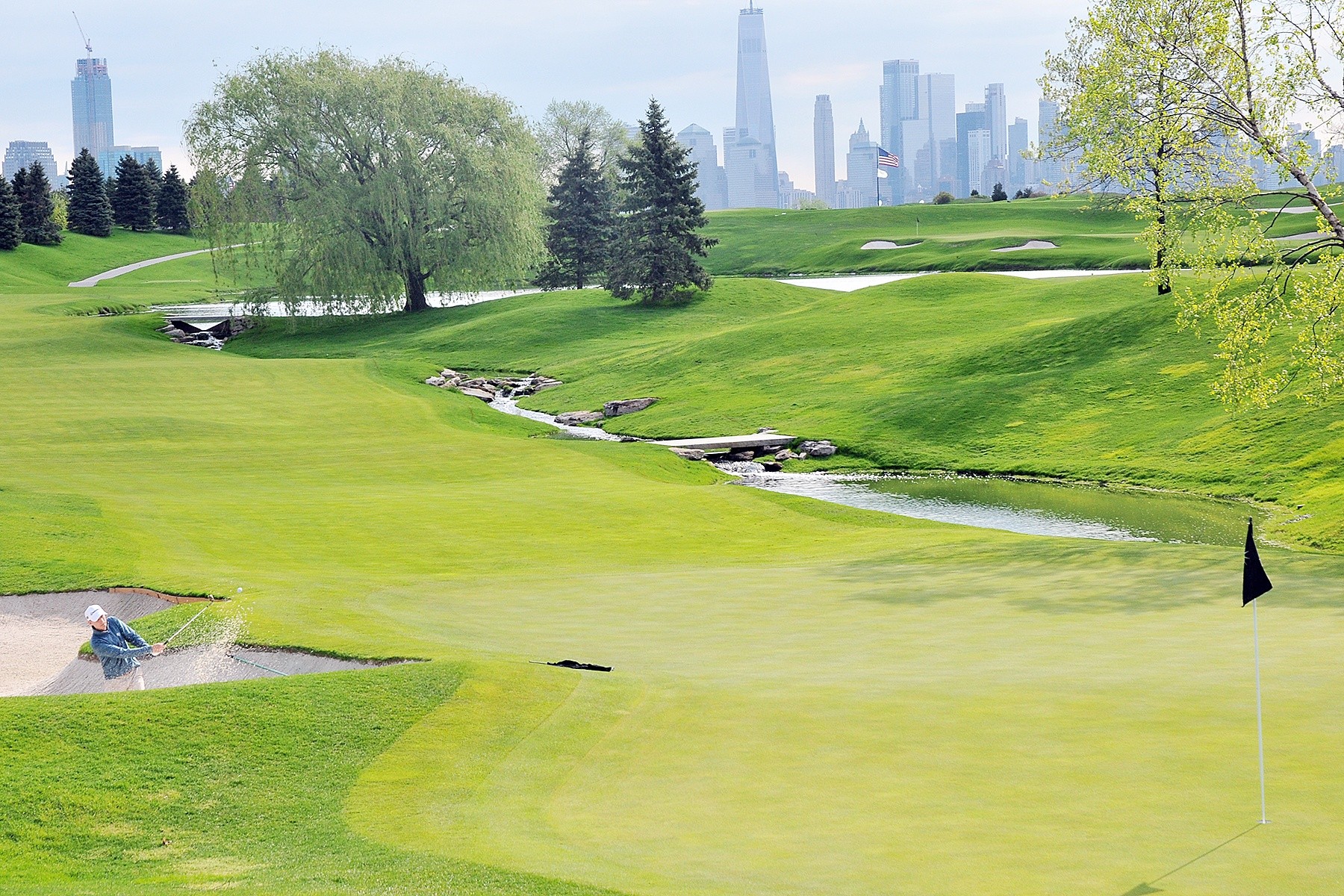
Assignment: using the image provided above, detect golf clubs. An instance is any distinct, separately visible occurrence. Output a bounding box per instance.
[149,593,215,658]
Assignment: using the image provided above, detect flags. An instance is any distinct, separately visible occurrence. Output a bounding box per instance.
[878,147,899,168]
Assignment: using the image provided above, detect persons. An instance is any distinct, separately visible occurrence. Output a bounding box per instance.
[84,604,166,692]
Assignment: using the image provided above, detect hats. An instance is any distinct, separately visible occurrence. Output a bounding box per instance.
[84,603,108,623]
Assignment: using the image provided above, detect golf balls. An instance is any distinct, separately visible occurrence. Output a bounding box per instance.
[237,587,242,593]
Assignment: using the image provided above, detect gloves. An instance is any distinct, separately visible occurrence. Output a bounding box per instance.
[150,650,164,656]
